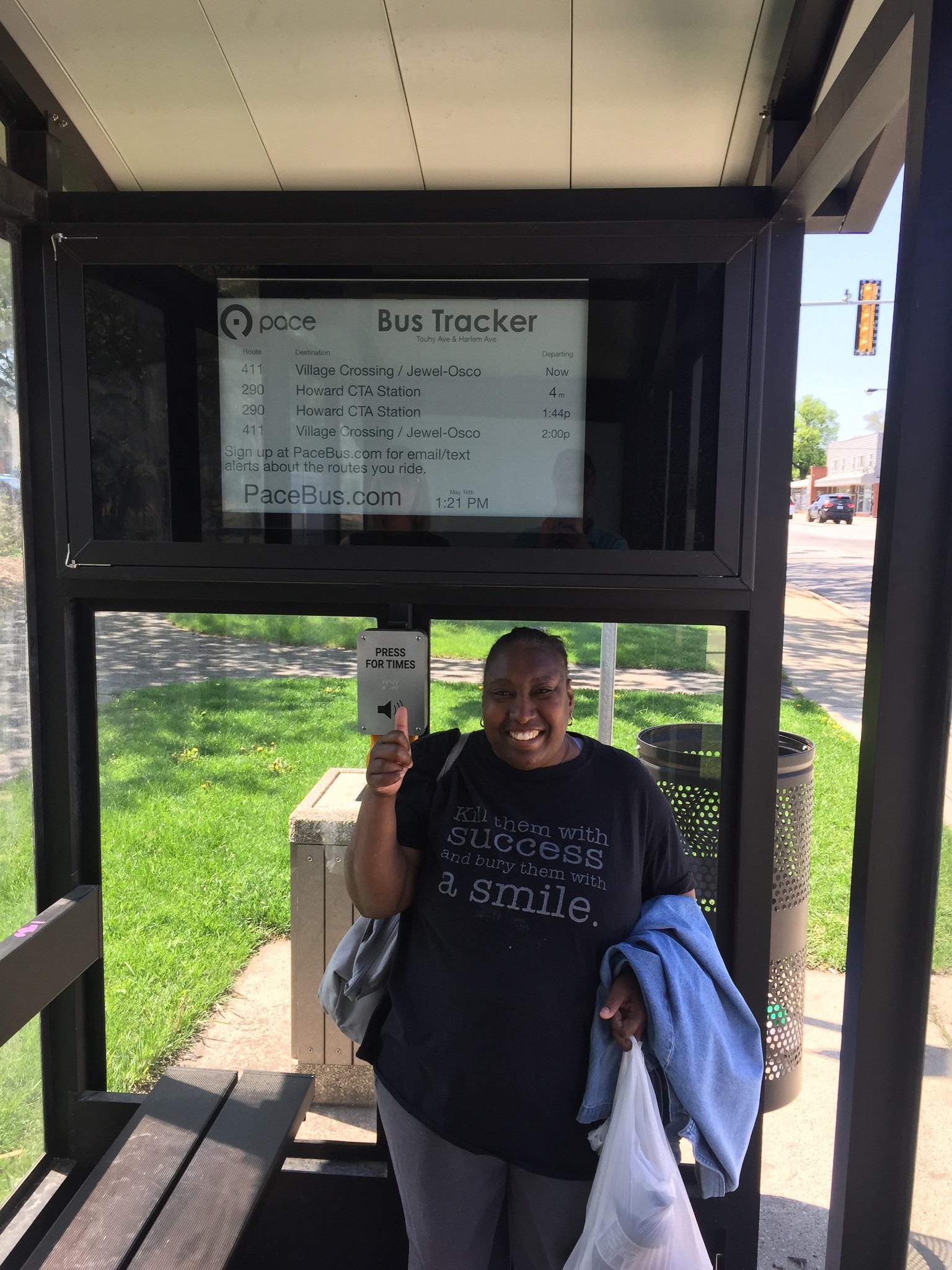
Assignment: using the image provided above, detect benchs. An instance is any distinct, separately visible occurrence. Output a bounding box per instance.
[0,884,316,1270]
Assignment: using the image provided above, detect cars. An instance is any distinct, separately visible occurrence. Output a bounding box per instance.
[789,496,796,519]
[0,474,23,505]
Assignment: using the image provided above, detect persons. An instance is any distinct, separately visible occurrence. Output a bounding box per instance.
[341,627,695,1270]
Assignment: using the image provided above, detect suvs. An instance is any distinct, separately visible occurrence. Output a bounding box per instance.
[806,493,854,524]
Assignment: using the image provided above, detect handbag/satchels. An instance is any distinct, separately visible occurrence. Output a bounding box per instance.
[562,1036,714,1269]
[319,913,398,1045]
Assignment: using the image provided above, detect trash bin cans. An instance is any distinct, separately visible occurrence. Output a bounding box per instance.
[637,726,815,1112]
